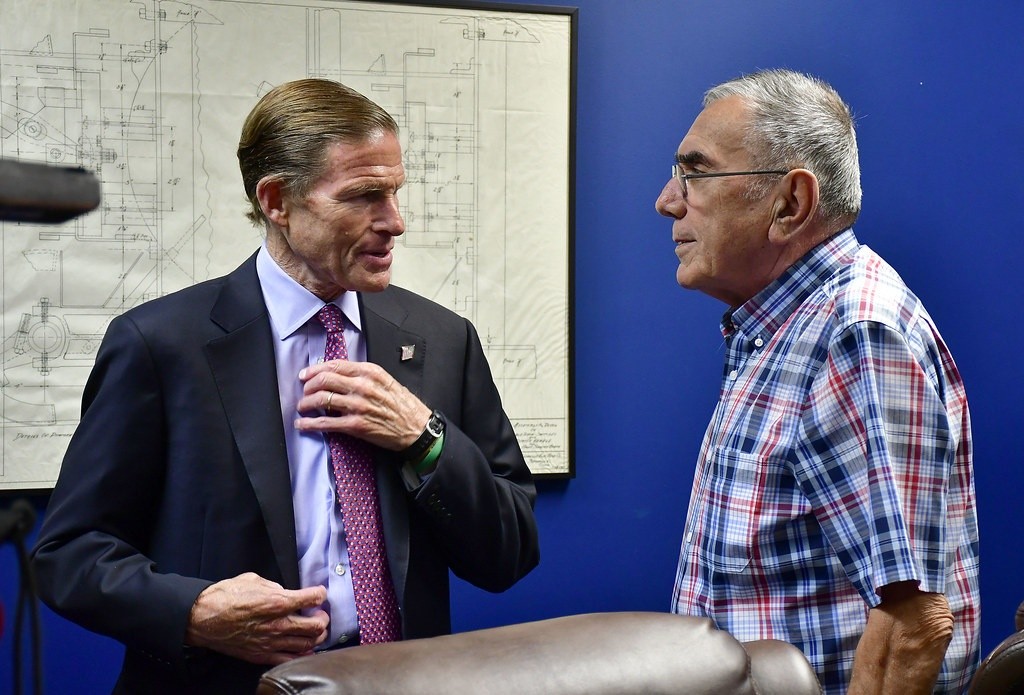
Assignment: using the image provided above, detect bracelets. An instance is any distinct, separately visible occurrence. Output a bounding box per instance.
[401,408,446,464]
[415,433,444,474]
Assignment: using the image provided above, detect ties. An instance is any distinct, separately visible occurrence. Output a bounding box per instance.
[318,308,402,643]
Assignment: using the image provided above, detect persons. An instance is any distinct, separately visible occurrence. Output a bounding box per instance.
[654,69,981,694]
[26,78,541,695]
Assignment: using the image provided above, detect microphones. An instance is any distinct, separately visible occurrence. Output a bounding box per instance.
[0,499,43,695]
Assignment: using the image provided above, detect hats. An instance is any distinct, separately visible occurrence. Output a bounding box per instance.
[1,159,100,229]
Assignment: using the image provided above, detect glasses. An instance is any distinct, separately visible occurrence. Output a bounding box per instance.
[671,164,790,198]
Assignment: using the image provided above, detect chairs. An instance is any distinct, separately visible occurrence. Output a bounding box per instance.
[257,610,827,695]
